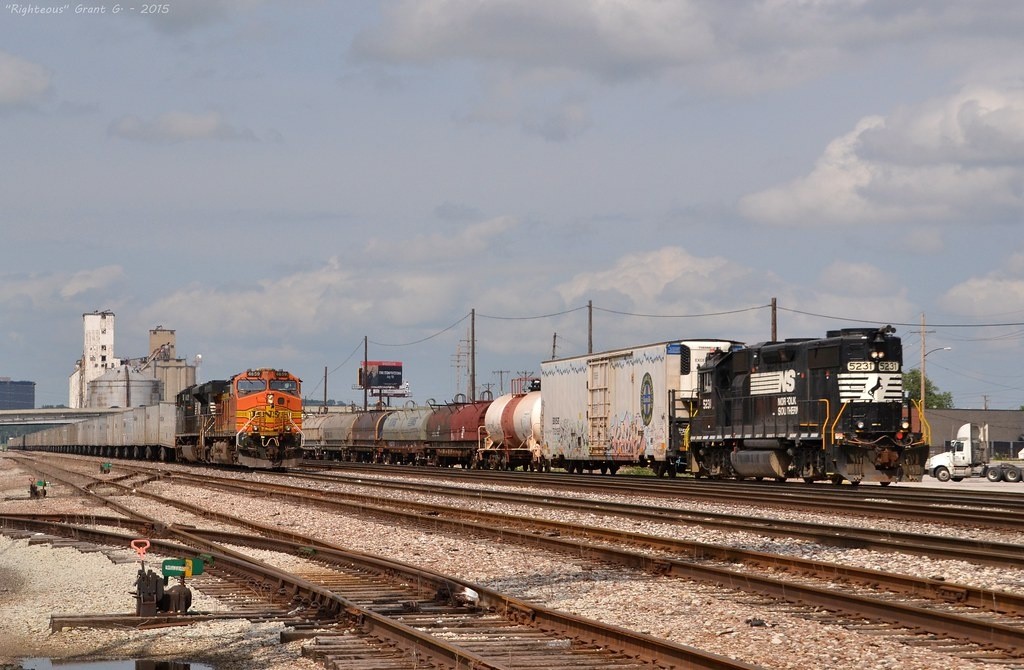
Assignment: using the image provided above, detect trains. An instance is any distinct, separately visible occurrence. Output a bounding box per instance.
[300,328,930,489]
[8,367,304,472]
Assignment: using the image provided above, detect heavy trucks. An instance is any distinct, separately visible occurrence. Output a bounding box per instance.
[924,423,1024,482]
